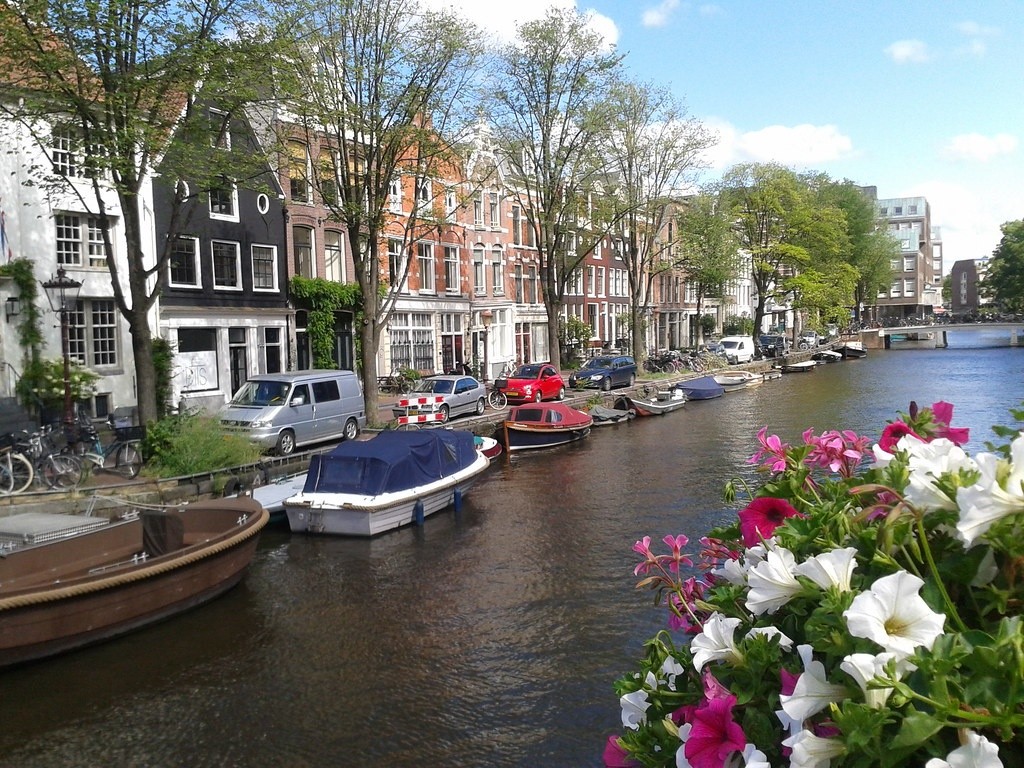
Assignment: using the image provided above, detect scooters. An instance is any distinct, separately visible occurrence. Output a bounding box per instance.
[447,359,474,378]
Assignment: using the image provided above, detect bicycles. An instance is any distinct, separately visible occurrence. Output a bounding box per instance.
[502,358,518,373]
[841,310,1023,333]
[480,378,508,410]
[641,349,710,374]
[0,414,145,495]
[376,369,412,397]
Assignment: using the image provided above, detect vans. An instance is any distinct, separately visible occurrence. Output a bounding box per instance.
[217,368,368,457]
[717,336,755,364]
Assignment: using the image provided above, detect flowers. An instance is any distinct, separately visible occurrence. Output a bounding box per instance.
[602,402,1024,768]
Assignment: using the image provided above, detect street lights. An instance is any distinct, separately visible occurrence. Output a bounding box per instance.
[41,264,84,456]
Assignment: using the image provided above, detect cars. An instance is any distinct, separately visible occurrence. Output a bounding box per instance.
[802,331,820,347]
[569,354,637,392]
[497,364,567,404]
[760,334,790,357]
[392,375,486,422]
[700,344,729,364]
[826,324,838,338]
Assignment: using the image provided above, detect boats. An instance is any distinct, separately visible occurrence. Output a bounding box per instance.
[627,368,782,413]
[1,489,270,665]
[502,402,638,453]
[889,334,908,343]
[229,426,501,541]
[772,342,868,375]
